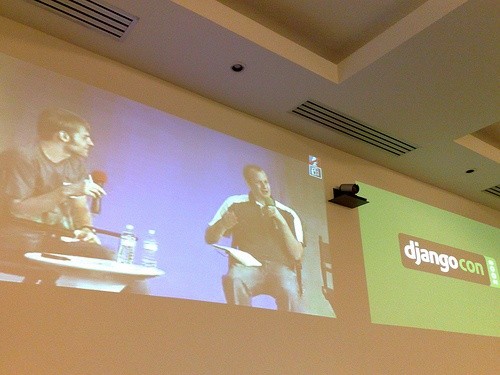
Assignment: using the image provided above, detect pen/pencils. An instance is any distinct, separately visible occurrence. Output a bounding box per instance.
[89,174,93,186]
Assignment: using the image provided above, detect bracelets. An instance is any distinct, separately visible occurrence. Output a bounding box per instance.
[80,226,97,234]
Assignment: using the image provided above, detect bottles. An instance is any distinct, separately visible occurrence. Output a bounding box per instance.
[141,228,160,268]
[117,224,136,263]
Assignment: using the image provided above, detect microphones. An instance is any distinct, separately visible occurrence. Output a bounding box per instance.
[264,196,279,231]
[90,170,106,215]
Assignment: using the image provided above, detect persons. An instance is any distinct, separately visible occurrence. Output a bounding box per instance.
[205,163,304,313]
[0,107,146,295]
